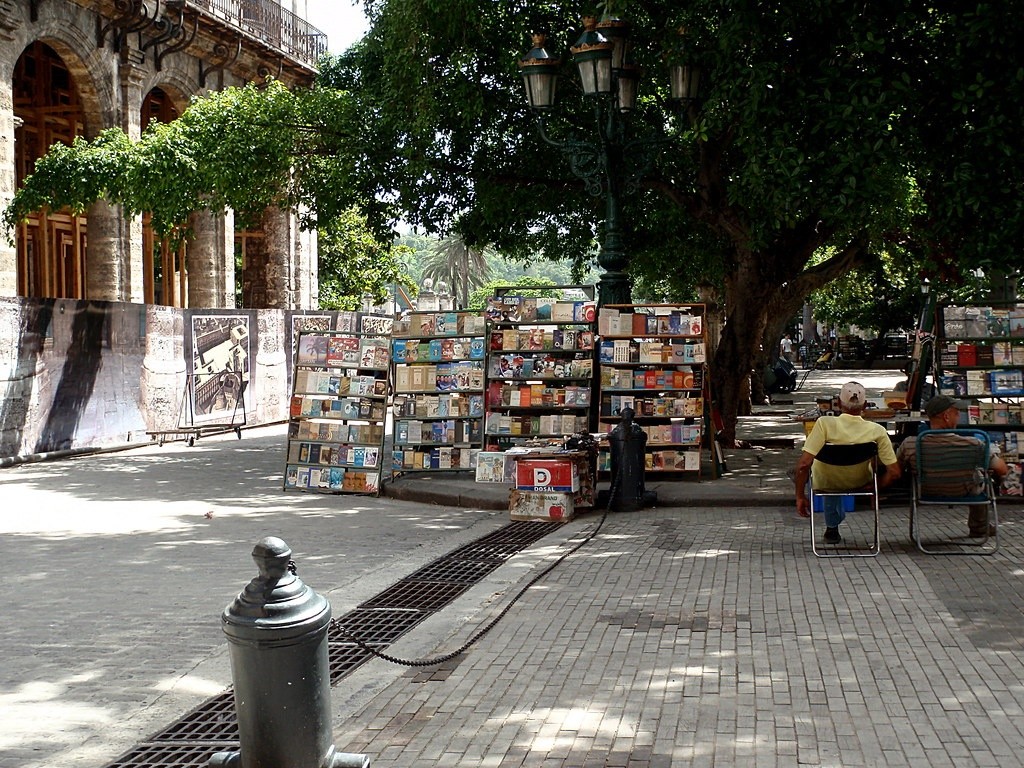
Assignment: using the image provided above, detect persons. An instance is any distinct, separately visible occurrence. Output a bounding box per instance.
[787,382,902,518]
[895,394,1007,536]
[774,328,833,391]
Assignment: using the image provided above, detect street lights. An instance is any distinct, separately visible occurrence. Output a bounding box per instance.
[517,17,701,314]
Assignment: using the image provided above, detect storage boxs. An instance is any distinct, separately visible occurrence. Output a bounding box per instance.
[508,489,575,523]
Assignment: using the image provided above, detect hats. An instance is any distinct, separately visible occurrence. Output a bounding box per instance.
[839,382,866,406]
[925,395,967,417]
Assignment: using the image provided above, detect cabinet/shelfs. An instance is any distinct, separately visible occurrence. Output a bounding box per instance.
[934,299,1024,502]
[282,283,727,499]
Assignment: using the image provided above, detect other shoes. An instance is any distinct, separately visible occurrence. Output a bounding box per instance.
[823,532,842,543]
[969,523,996,537]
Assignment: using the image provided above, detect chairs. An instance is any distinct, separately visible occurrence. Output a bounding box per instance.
[808,440,880,557]
[909,429,1000,556]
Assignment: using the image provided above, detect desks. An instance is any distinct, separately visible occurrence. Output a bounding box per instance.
[794,396,930,439]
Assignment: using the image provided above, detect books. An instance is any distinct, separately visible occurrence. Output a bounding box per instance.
[289,273,704,503]
[930,291,1023,498]
